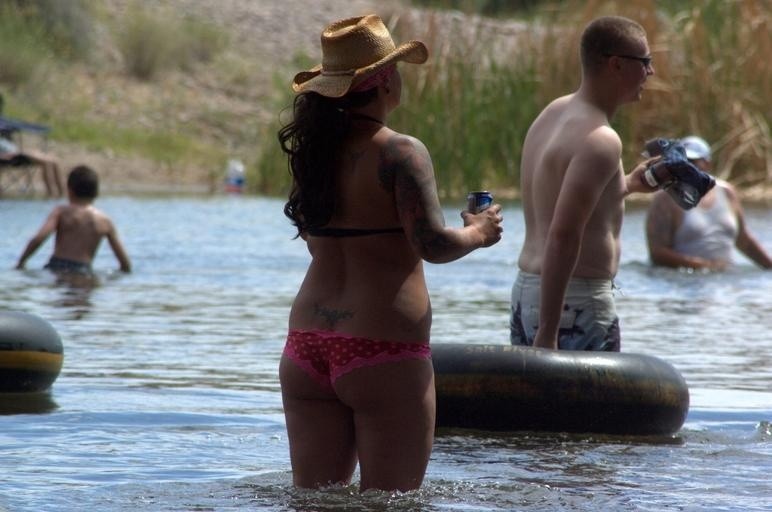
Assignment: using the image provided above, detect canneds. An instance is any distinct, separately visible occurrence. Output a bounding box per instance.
[467,191,494,215]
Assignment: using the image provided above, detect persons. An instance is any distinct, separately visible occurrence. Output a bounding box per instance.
[0,135,66,199]
[646,136,772,272]
[278,15,504,495]
[16,165,131,276]
[509,16,678,353]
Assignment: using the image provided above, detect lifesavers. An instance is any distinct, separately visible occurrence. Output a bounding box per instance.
[428,343,690,436]
[0,309,64,392]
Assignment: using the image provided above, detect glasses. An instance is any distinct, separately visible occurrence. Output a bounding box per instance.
[614,53,654,66]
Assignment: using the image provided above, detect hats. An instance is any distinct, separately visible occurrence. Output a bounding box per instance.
[291,13,429,98]
[682,133,715,166]
